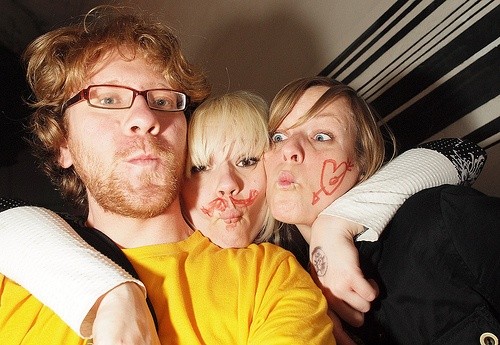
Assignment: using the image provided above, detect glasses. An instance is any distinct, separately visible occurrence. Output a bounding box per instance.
[60,84,192,117]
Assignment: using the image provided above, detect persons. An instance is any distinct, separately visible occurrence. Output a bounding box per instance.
[0,8,339,345]
[0,87,487,345]
[263,74,500,345]
